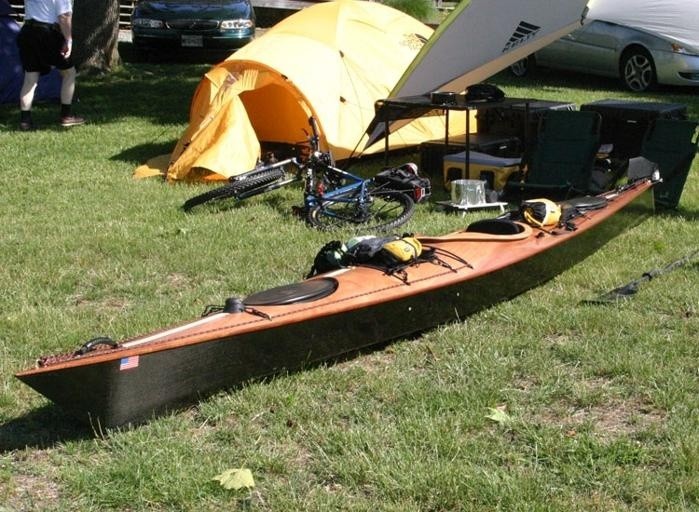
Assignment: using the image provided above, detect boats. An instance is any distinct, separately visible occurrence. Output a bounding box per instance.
[10,166,662,431]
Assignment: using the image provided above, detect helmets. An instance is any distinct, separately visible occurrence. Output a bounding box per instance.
[315,240,349,273]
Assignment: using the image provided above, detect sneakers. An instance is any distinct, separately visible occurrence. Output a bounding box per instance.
[20,119,32,130]
[61,115,86,127]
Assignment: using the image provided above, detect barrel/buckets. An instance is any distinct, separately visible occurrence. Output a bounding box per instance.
[451,178,487,205]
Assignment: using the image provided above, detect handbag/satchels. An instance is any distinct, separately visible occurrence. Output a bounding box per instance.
[344,236,434,273]
[519,198,562,227]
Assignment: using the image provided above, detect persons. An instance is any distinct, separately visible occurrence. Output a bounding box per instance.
[16,0,88,131]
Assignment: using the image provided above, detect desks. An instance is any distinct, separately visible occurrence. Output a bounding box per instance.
[375,93,538,180]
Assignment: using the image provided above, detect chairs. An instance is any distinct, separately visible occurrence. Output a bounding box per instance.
[505,109,603,200]
[607,118,699,213]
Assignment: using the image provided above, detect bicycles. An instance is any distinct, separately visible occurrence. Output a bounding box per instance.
[182,116,430,234]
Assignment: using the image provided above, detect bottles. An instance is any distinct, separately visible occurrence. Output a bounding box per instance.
[592,153,611,184]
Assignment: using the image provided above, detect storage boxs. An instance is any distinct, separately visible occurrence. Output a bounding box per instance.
[579,97,687,145]
[442,149,521,195]
[476,97,576,140]
[420,134,521,177]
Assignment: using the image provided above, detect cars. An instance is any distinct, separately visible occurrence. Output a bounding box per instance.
[131,1,257,58]
[506,15,699,93]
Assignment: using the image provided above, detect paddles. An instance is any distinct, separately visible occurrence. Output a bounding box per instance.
[581,249,699,304]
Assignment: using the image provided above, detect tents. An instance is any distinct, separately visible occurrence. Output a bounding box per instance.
[162,1,478,185]
